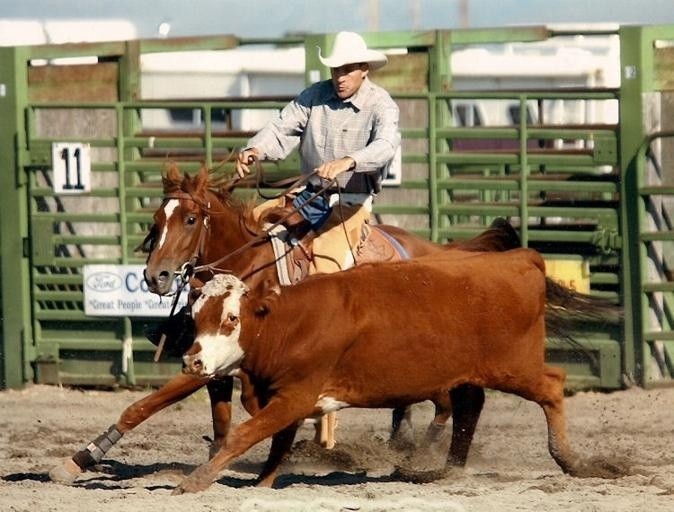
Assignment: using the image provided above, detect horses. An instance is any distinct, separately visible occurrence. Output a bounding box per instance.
[48,146,624,485]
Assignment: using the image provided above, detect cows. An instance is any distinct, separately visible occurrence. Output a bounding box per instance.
[170,247,629,497]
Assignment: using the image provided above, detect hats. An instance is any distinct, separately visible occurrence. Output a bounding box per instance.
[319,31,388,70]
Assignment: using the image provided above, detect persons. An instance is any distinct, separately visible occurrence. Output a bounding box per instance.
[237,30,400,275]
